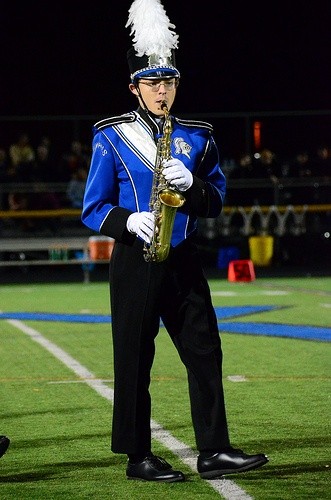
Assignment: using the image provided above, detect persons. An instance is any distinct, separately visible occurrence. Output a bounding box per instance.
[83,43,276,484]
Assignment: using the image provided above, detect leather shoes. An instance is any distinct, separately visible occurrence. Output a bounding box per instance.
[125,453,185,484]
[197,445,270,481]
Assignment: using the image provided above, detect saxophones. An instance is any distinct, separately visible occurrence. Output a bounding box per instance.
[144,98,185,263]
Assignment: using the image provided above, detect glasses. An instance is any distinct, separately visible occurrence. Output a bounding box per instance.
[137,78,181,92]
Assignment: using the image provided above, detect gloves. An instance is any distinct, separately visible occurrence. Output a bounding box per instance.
[162,156,194,192]
[127,210,159,245]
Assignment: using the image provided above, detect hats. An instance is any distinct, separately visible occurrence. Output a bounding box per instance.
[126,0,181,83]
